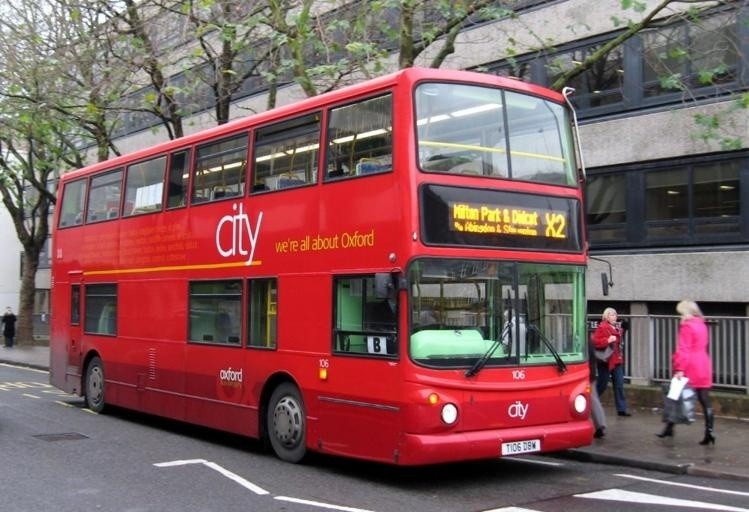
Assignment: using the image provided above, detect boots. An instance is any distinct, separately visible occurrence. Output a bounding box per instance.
[699,408,715,446]
[654,423,673,437]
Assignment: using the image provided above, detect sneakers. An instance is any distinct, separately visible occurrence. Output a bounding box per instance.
[617,411,631,417]
[593,426,607,439]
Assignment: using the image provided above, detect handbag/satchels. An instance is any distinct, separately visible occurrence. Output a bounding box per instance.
[661,383,697,424]
[592,343,613,364]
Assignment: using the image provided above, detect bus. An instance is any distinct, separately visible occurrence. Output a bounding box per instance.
[48,65,614,469]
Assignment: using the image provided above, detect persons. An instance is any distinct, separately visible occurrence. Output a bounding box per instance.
[371,272,401,353]
[652,299,719,447]
[1,308,18,349]
[564,317,608,441]
[591,307,635,418]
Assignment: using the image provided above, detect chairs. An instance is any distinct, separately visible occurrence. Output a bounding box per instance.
[364,301,395,334]
[61,157,397,225]
